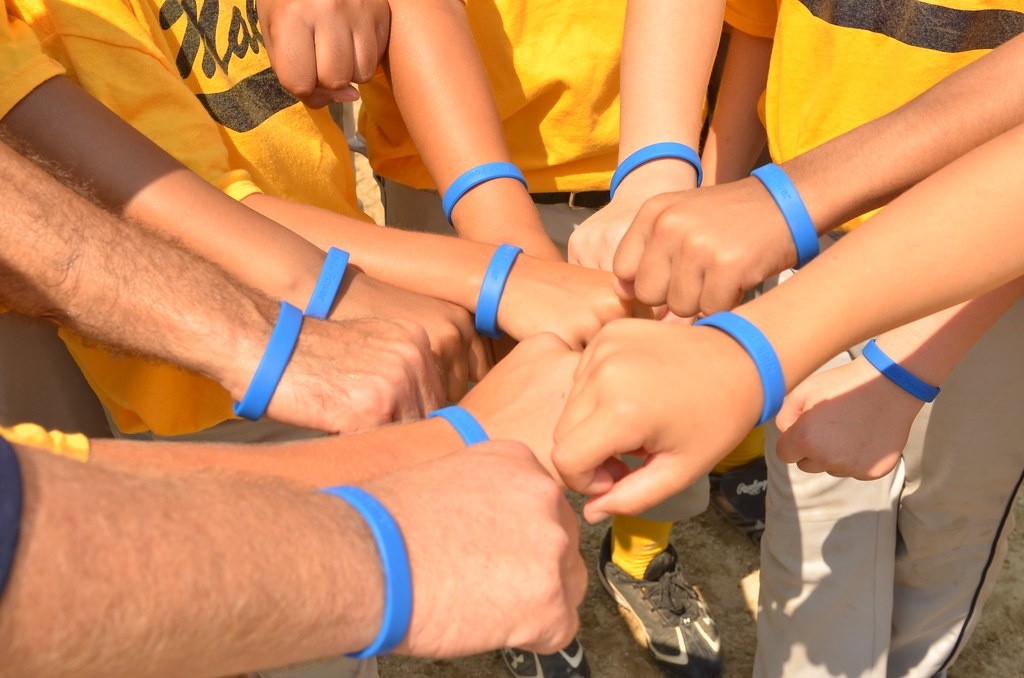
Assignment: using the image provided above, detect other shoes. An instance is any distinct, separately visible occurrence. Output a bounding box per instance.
[708,454,768,549]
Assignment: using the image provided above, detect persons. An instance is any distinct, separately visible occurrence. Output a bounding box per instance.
[1,1,1021,678]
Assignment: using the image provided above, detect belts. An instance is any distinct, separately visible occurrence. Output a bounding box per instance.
[529,190,610,211]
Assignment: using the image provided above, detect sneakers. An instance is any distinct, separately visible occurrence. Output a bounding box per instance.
[595,520,724,678]
[500,633,592,678]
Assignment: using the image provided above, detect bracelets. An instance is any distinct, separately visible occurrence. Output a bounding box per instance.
[441,162,528,230]
[233,301,303,421]
[426,406,491,447]
[610,142,704,200]
[304,246,349,319]
[750,164,820,270]
[318,484,411,658]
[693,311,784,429]
[475,243,524,338]
[863,340,941,404]
